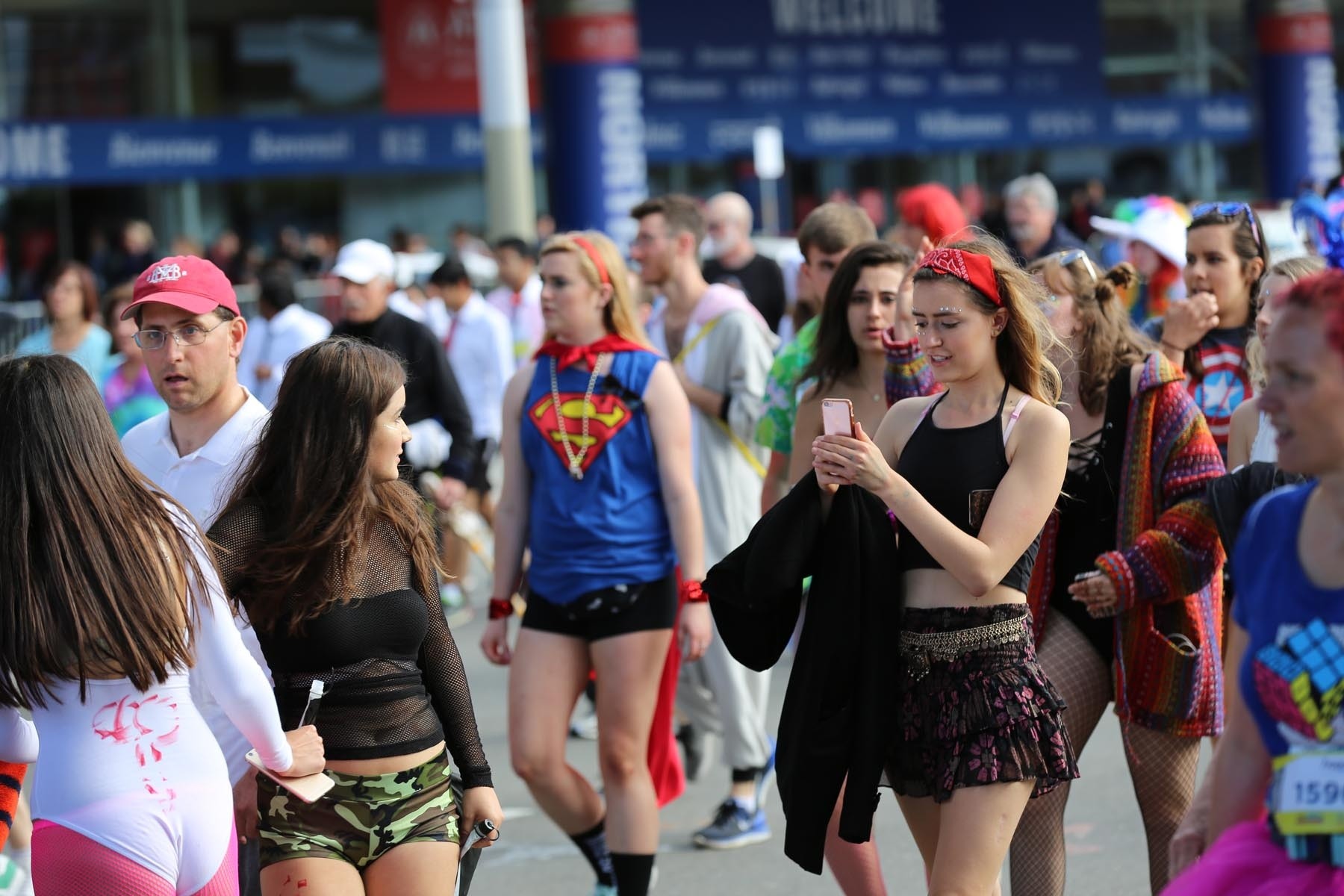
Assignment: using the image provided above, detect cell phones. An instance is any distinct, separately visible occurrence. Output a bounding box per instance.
[821,397,854,476]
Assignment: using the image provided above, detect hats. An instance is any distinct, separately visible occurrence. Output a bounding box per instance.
[1091,208,1187,273]
[330,239,397,285]
[121,255,240,321]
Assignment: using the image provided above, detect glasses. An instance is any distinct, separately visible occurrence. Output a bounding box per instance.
[1192,202,1266,245]
[131,319,224,350]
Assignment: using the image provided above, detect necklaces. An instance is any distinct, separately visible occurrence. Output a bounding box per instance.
[1199,339,1244,427]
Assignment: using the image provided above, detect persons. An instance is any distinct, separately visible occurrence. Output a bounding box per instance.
[550,351,604,480]
[0,173,1344,896]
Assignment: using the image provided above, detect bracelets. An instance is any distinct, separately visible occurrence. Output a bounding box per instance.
[1160,338,1187,353]
[488,597,513,620]
[682,579,710,602]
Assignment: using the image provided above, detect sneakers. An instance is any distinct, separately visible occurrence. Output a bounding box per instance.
[756,747,780,798]
[693,796,768,847]
[678,725,716,784]
[572,708,602,740]
